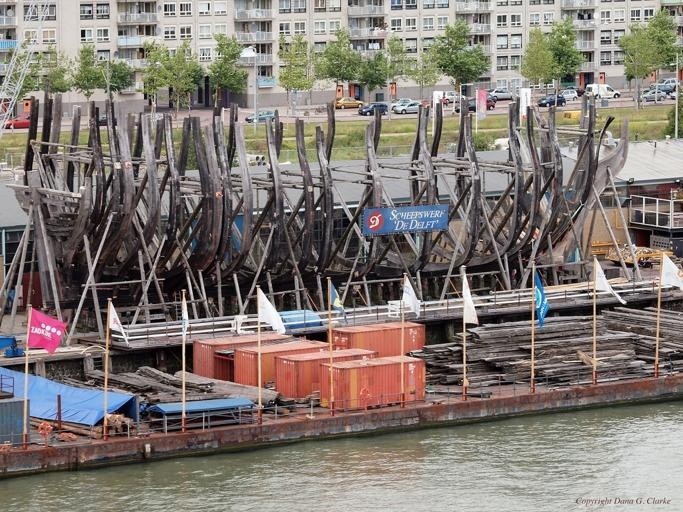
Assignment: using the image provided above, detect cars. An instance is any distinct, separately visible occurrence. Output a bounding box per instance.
[246,109,278,121]
[98,112,117,126]
[5,113,31,129]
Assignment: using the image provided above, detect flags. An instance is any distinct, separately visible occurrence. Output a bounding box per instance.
[534,271,549,328]
[660,253,682,291]
[402,276,420,319]
[256,288,286,335]
[595,259,626,305]
[329,281,348,325]
[181,296,188,337]
[27,308,65,353]
[462,274,478,325]
[108,302,128,347]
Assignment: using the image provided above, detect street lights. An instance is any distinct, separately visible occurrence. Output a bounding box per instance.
[239,46,260,137]
